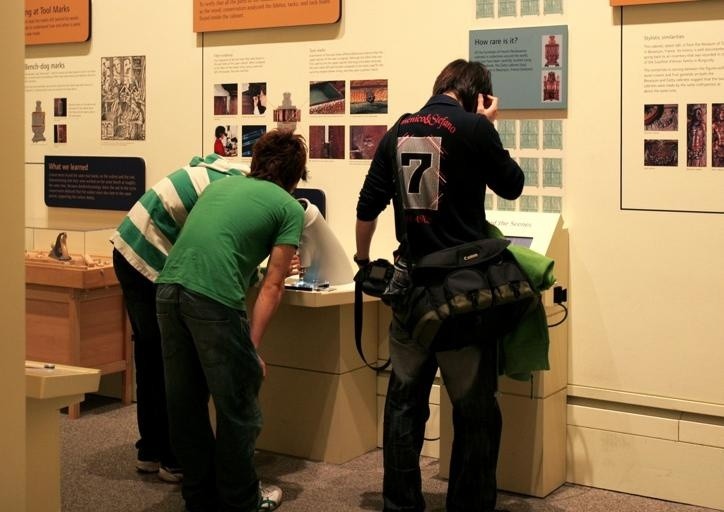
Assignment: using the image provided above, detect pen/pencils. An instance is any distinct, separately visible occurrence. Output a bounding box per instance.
[25,363,56,369]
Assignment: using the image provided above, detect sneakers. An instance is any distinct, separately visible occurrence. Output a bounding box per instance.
[136,445,161,471]
[256,480,282,512]
[157,461,186,482]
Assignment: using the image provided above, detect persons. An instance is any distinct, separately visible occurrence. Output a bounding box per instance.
[154,125,311,512]
[109,153,251,482]
[253,85,267,115]
[229,137,237,156]
[212,125,226,157]
[354,60,524,512]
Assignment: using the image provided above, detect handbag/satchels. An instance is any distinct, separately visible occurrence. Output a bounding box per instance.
[393,236,539,351]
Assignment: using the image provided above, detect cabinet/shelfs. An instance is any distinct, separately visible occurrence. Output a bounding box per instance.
[439,231,569,499]
[26,251,136,419]
[207,282,380,465]
